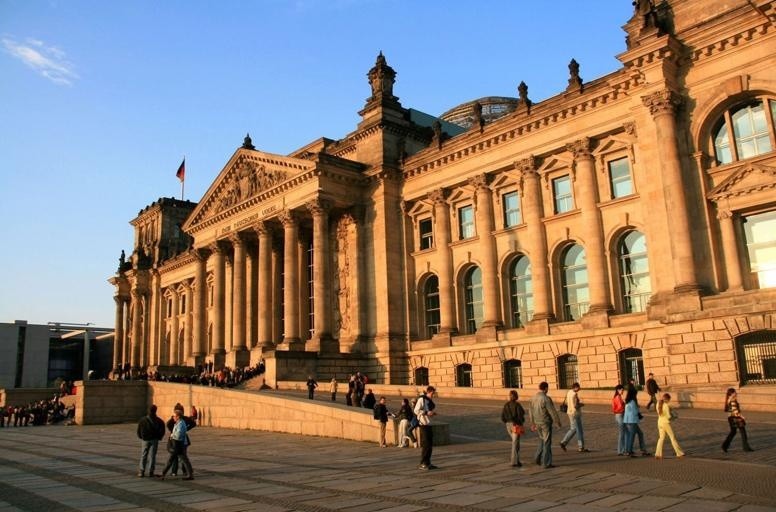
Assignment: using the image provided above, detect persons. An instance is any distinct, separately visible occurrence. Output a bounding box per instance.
[612,384,626,456]
[0,379,75,427]
[373,396,391,448]
[655,392,686,459]
[136,404,165,478]
[560,381,590,452]
[646,371,662,409]
[623,387,651,457]
[626,378,645,419]
[407,397,419,447]
[112,361,266,389]
[154,410,194,480]
[119,249,125,267]
[167,402,196,477]
[306,375,318,399]
[328,377,338,402]
[720,387,756,453]
[528,381,562,469]
[393,398,414,448]
[414,385,439,471]
[345,370,377,409]
[502,390,526,467]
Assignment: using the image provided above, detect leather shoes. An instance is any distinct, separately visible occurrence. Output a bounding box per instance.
[722,447,727,452]
[137,470,194,481]
[509,459,561,468]
[746,449,755,452]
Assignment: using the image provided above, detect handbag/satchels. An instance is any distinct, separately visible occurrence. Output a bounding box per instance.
[733,418,746,427]
[167,436,187,455]
[512,422,526,435]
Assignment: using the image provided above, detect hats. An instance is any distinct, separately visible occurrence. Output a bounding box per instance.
[174,403,184,414]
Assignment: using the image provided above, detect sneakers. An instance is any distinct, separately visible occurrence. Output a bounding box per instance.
[560,443,567,452]
[429,466,437,470]
[616,449,686,459]
[578,447,592,452]
[381,441,418,448]
[417,464,429,471]
[560,403,568,413]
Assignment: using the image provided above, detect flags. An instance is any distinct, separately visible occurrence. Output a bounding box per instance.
[175,159,185,185]
